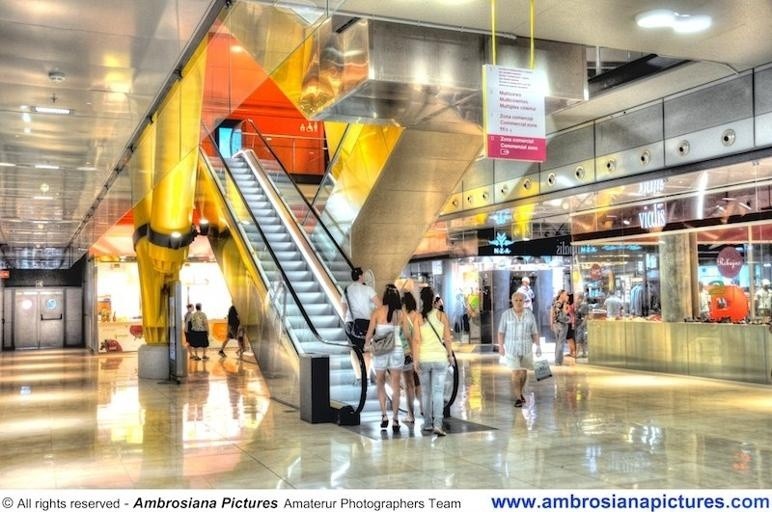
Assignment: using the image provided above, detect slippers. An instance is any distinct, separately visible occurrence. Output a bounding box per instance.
[521,394,525,403]
[514,399,522,407]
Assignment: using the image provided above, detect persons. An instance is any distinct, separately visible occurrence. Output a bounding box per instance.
[401,292,424,423]
[698,280,713,321]
[576,292,588,357]
[550,289,569,367]
[604,291,624,319]
[753,276,772,317]
[517,277,536,313]
[236,323,246,358]
[190,302,210,361]
[363,284,410,431]
[182,304,195,359]
[434,297,442,310]
[218,305,240,358]
[341,267,382,385]
[498,292,543,408]
[566,293,578,358]
[412,287,452,436]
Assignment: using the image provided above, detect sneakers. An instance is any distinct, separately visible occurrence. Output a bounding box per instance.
[190,355,209,360]
[219,351,226,357]
[380,415,447,437]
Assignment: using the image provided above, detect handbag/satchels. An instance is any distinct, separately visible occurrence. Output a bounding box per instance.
[558,312,571,324]
[372,330,396,356]
[350,319,370,340]
[534,360,552,381]
